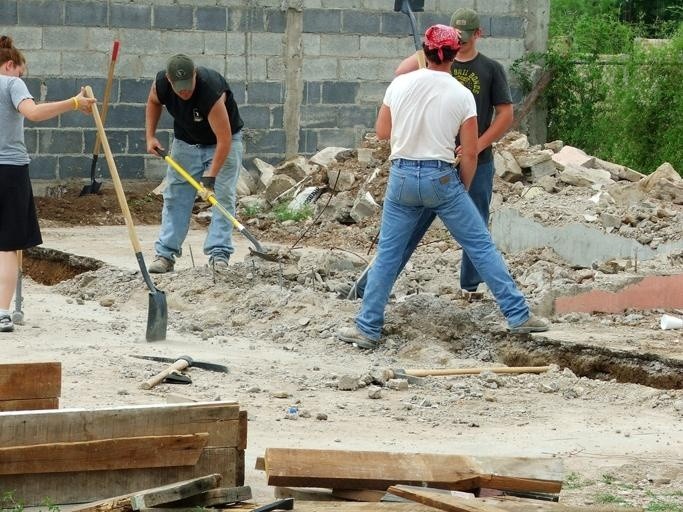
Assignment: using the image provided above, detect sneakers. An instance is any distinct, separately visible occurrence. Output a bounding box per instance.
[337,326,374,348]
[149,255,173,272]
[209,252,227,266]
[0,314,14,331]
[505,312,548,335]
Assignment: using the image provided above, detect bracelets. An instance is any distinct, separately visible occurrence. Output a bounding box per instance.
[68,96,79,112]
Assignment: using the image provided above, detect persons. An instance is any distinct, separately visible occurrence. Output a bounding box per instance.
[143,51,249,276]
[0,38,98,331]
[336,7,516,301]
[336,24,550,348]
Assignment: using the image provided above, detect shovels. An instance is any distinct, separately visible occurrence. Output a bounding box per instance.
[155,147,278,262]
[85,84,168,342]
[394,0,426,69]
[80,40,120,197]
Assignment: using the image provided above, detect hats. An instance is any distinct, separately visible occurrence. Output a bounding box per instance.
[166,55,194,91]
[451,7,479,42]
